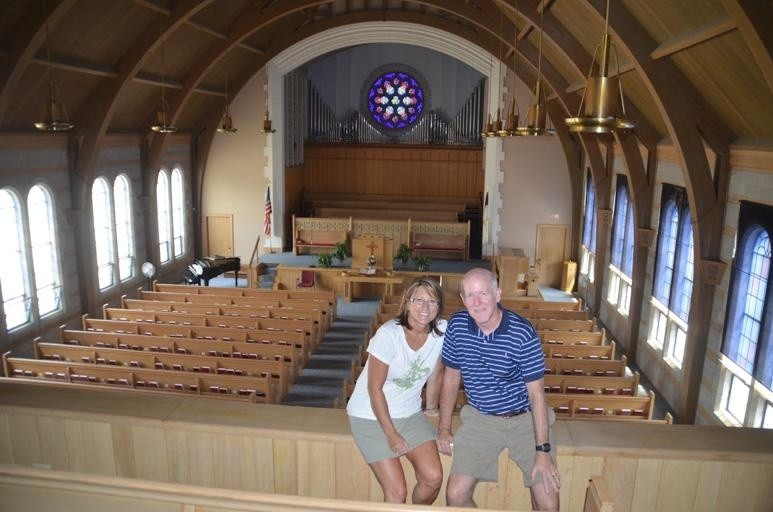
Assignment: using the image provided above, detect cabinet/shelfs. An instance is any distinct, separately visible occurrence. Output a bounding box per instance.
[496,247,530,293]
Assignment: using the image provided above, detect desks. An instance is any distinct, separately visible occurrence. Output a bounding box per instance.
[334,274,403,303]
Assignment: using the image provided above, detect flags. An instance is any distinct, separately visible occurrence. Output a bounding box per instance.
[263,186,273,238]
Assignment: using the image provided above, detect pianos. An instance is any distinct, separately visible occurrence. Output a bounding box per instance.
[185,257,239,287]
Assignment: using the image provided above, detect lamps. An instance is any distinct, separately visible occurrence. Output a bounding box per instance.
[258,0,276,133]
[150,1,177,134]
[32,0,74,132]
[481,1,633,138]
[216,0,238,133]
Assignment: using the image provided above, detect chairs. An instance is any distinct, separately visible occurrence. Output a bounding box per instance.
[295,271,316,292]
[427,275,443,290]
[527,260,541,290]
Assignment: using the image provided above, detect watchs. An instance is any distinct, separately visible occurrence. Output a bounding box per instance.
[536,443,551,453]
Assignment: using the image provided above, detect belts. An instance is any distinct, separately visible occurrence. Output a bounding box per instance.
[496,409,532,417]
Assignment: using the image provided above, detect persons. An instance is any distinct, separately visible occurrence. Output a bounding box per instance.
[347,277,448,506]
[435,267,560,512]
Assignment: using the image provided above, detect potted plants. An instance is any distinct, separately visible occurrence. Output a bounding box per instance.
[334,242,344,264]
[415,255,432,272]
[396,243,411,265]
[319,254,331,267]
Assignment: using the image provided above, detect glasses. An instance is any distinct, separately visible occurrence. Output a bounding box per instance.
[411,297,439,305]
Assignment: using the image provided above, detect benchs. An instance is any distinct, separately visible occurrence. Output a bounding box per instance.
[296,229,348,255]
[2,279,336,404]
[334,293,673,425]
[224,264,250,279]
[413,233,468,261]
